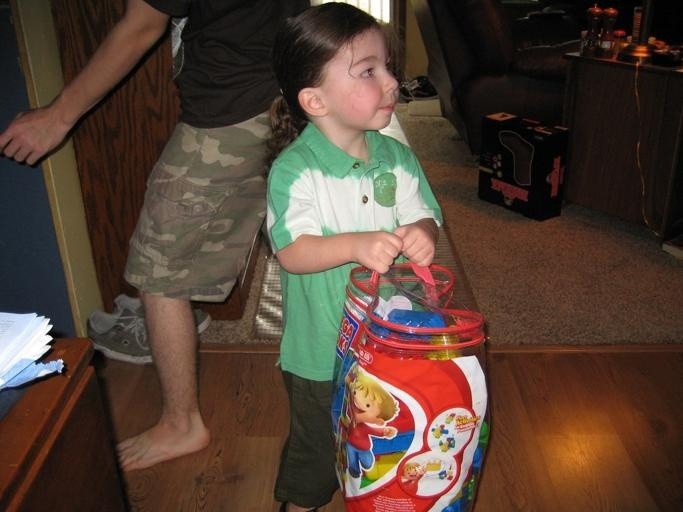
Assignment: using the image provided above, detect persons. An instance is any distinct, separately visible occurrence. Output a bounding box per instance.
[0,0,307,474]
[257,1,446,512]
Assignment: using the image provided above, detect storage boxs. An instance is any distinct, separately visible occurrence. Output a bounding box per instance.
[477,110,566,221]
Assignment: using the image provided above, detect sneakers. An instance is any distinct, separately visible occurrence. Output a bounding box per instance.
[86,294,212,365]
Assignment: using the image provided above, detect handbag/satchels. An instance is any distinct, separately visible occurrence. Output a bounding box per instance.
[332,265,491,512]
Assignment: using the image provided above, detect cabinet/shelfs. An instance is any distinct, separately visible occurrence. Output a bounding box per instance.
[562,45,683,243]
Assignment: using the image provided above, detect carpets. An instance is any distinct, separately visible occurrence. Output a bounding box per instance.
[252,220,480,343]
